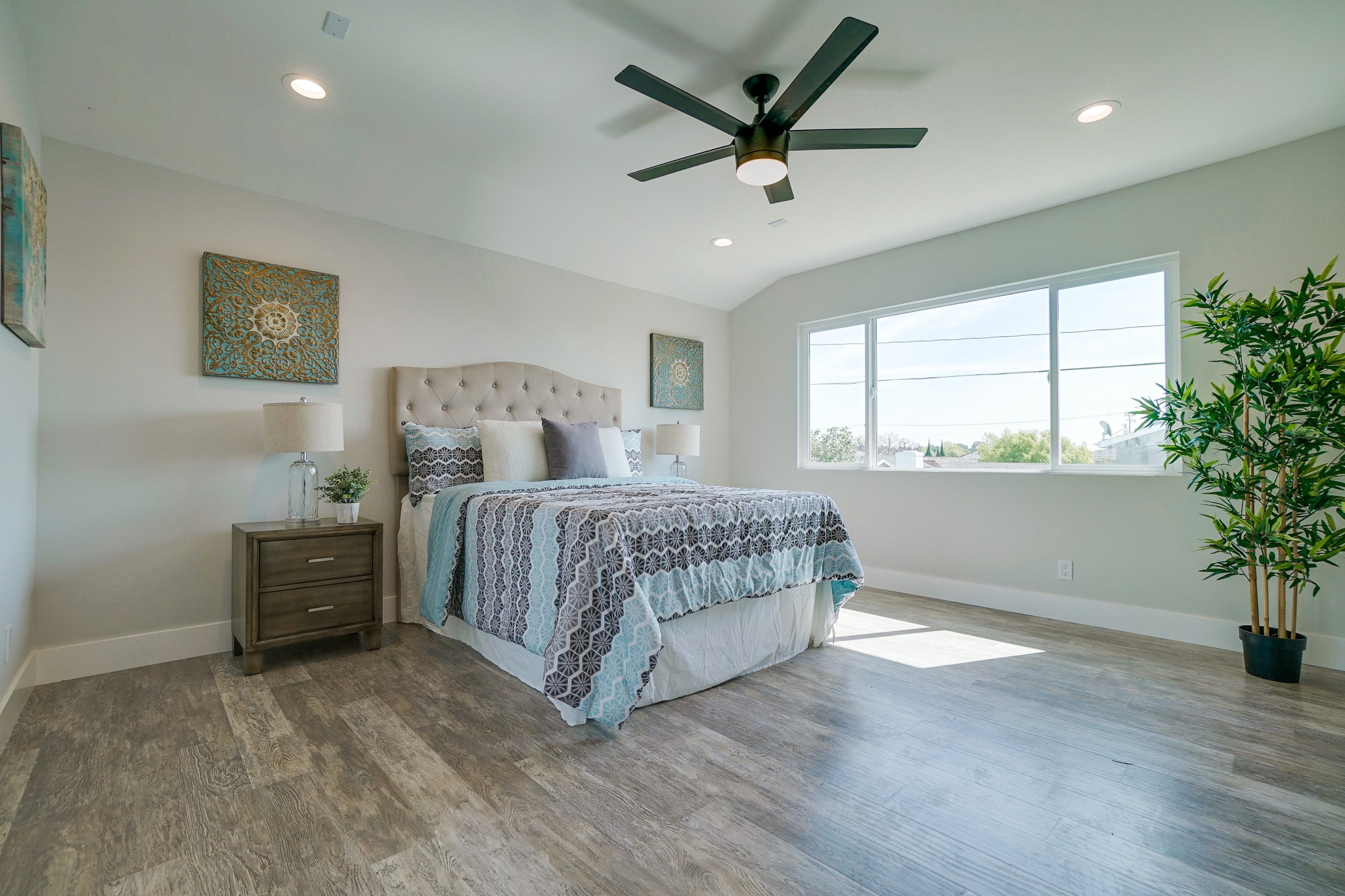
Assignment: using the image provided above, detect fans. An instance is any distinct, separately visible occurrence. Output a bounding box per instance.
[614,17,928,204]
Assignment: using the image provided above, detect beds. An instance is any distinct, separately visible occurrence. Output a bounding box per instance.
[389,361,865,730]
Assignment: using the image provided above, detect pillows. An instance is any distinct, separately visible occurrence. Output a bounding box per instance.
[399,417,641,507]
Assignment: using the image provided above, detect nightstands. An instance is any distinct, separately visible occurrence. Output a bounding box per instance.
[232,517,384,674]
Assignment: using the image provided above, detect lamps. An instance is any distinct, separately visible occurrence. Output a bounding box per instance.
[735,125,790,186]
[262,397,344,524]
[656,421,700,478]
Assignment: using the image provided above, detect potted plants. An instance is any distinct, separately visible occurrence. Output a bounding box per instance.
[313,463,378,524]
[1127,252,1345,683]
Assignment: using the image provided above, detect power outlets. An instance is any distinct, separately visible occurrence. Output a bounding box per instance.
[1058,559,1073,580]
